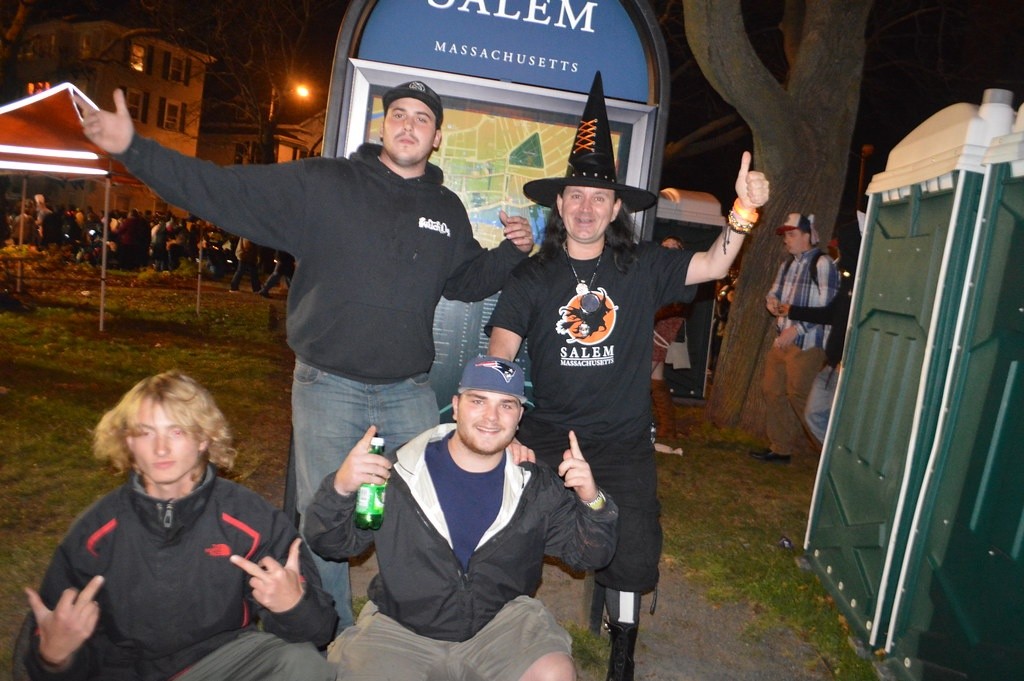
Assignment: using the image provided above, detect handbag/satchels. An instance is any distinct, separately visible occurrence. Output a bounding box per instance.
[664,342,691,369]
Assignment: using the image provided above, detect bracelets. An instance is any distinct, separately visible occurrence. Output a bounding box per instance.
[720,198,759,255]
[582,490,607,510]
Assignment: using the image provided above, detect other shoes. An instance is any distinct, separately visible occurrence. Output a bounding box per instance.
[230,289,242,294]
[258,290,272,298]
[750,449,792,465]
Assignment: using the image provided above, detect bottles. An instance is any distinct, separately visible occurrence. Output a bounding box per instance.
[355,436,387,531]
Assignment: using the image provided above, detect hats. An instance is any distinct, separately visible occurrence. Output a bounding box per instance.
[457,356,528,405]
[655,232,685,246]
[776,213,811,235]
[523,71,658,215]
[382,81,443,120]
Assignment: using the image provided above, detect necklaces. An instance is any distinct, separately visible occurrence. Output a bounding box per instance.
[564,241,606,336]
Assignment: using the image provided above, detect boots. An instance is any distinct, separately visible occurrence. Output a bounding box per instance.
[650,378,677,440]
[603,618,639,681]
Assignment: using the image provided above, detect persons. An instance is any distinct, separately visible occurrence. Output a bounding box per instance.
[228,237,265,296]
[651,238,686,439]
[483,150,773,681]
[259,249,295,297]
[0,194,239,282]
[777,275,854,446]
[13,372,341,681]
[304,355,620,681]
[746,212,839,465]
[69,82,536,637]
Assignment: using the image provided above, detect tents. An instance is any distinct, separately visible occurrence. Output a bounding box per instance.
[0,81,206,333]
